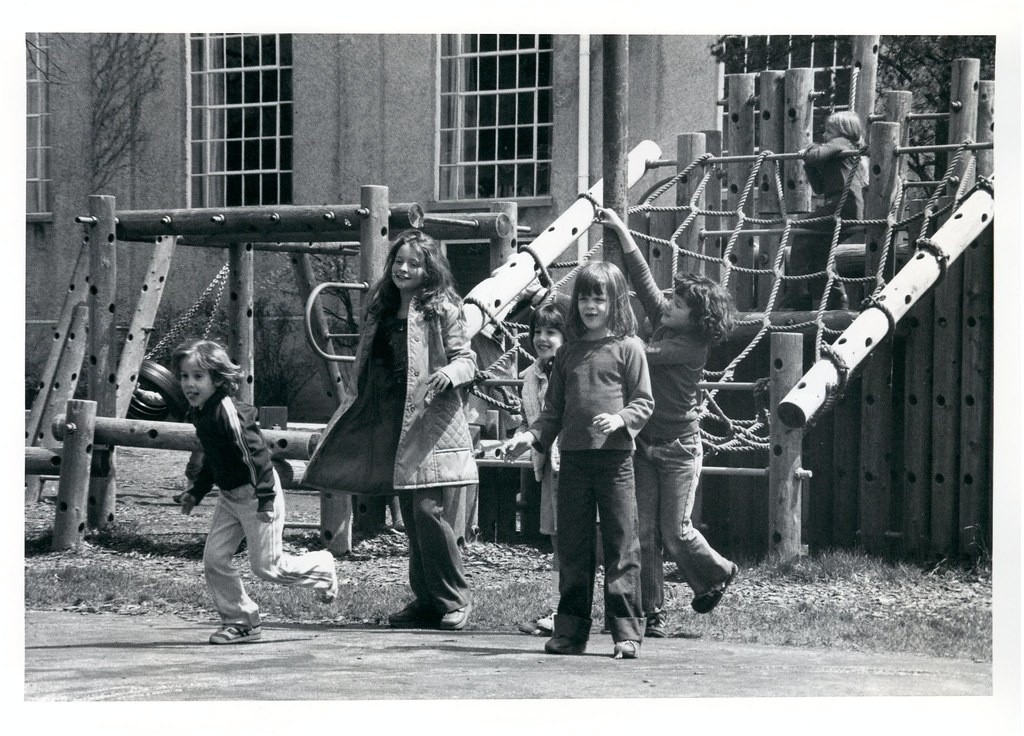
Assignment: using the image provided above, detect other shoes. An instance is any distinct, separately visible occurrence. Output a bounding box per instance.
[441,601,472,629]
[519,609,554,633]
[645,612,666,638]
[545,636,586,654]
[389,603,441,628]
[536,612,557,632]
[615,641,641,658]
[691,563,739,614]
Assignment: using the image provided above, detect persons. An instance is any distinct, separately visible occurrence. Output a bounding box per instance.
[172,338,338,644]
[593,207,738,638]
[513,303,580,635]
[501,262,655,658]
[776,111,868,311]
[301,228,480,630]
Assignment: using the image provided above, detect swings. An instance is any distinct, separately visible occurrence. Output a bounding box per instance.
[128,261,230,421]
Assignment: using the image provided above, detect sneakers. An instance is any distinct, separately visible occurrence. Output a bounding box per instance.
[314,552,338,604]
[209,625,261,644]
